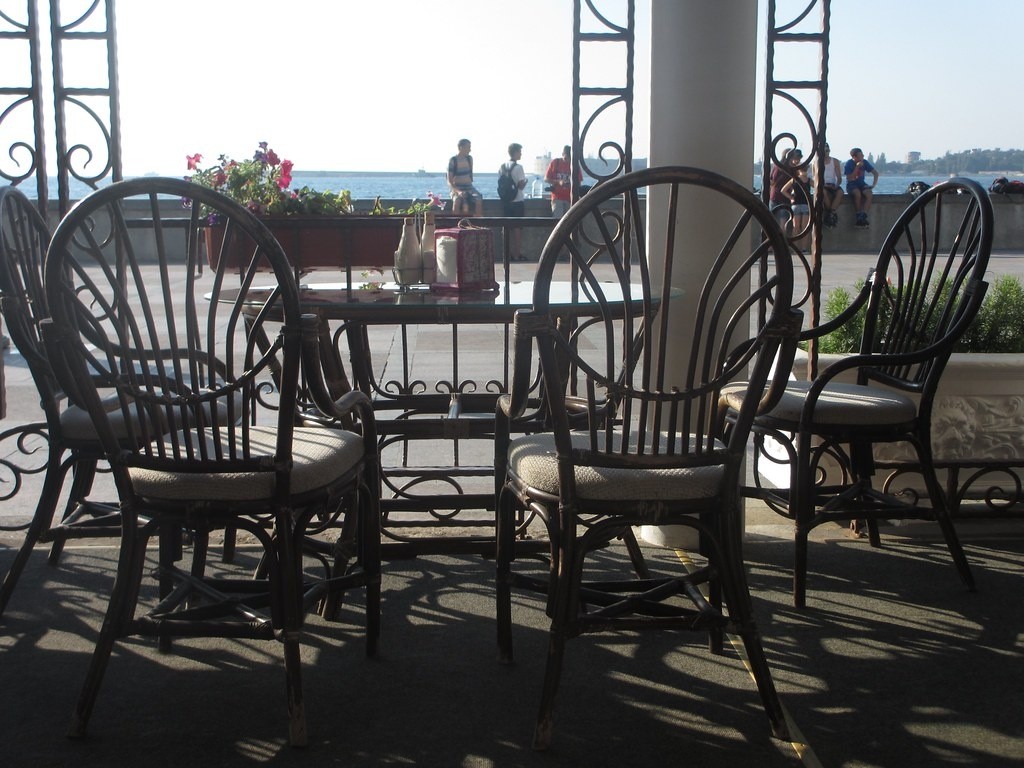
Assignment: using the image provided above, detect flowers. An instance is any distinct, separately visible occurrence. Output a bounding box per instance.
[181,143,446,226]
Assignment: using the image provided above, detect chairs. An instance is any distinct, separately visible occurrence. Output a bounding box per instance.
[493,166,804,754]
[0,179,383,749]
[700,177,995,611]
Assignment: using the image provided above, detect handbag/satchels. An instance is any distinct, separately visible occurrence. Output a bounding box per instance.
[908,177,1023,195]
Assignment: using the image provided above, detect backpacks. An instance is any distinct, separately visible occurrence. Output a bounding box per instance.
[496,163,520,202]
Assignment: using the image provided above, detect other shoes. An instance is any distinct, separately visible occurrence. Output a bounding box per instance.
[854,220,870,229]
[825,213,837,229]
[801,248,811,255]
[514,254,528,261]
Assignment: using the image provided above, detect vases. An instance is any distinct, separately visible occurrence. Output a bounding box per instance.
[204,213,475,274]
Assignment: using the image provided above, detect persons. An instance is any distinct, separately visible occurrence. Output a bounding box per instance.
[812,143,843,229]
[844,148,879,229]
[781,167,814,254]
[447,139,482,216]
[498,143,528,260]
[544,145,583,261]
[768,148,803,252]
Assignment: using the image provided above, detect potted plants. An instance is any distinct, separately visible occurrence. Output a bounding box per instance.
[757,269,1024,492]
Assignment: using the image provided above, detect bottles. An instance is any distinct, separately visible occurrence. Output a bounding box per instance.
[394,217,422,284]
[419,211,436,284]
[532,177,542,198]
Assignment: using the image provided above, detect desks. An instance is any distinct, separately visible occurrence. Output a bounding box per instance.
[204,281,684,618]
